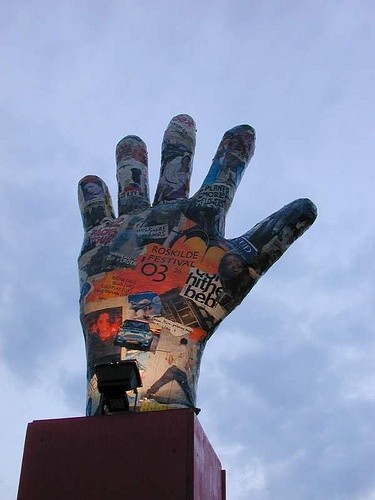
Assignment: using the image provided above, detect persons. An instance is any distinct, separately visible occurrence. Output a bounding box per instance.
[77,113,318,416]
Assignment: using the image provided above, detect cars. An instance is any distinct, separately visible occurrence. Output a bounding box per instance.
[115,319,154,348]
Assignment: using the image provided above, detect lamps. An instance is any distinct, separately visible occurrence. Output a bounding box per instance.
[94,358,143,414]
